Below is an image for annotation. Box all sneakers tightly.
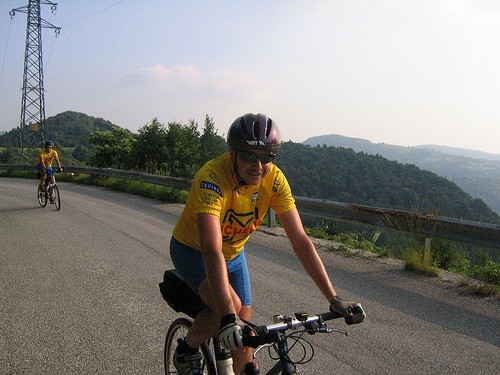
[39,183,46,197]
[172,338,205,374]
[50,198,56,204]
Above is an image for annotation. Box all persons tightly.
[37,141,63,205]
[170,113,367,375]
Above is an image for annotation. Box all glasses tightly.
[241,153,279,165]
[47,146,52,148]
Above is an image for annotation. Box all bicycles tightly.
[158,269,363,375]
[36,169,61,211]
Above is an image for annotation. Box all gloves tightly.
[58,168,62,172]
[42,168,48,173]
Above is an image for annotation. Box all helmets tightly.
[228,113,282,153]
[46,140,54,150]
